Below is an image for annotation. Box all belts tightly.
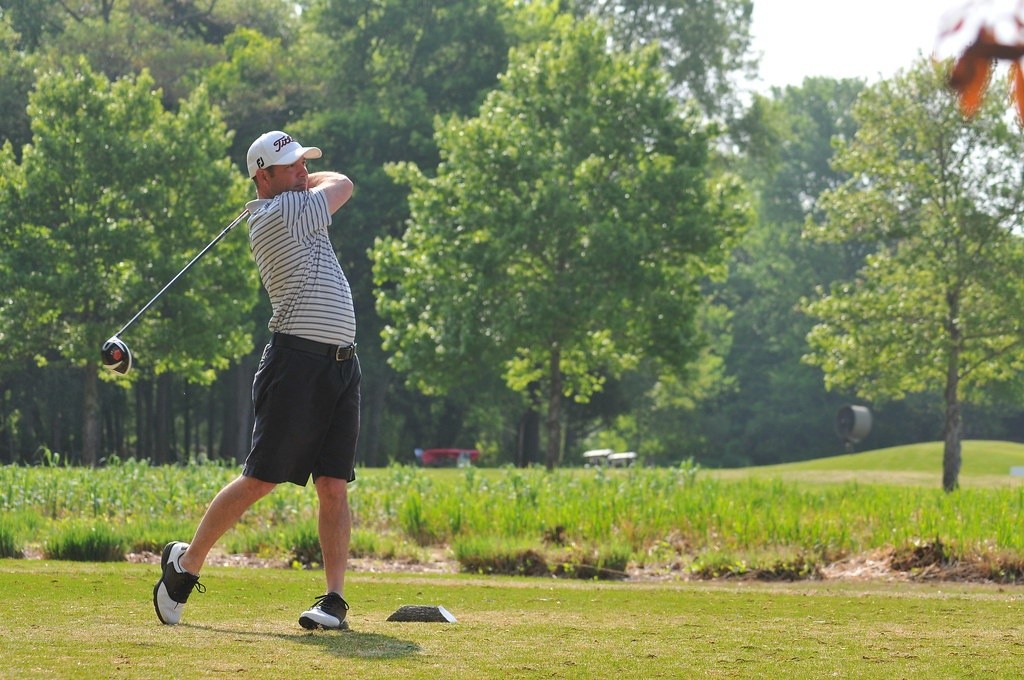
[269,333,358,361]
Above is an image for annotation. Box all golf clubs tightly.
[98,205,250,377]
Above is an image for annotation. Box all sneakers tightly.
[153,540,206,625]
[298,592,350,630]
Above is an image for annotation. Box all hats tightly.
[247,130,322,178]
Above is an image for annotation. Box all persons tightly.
[151,131,362,628]
